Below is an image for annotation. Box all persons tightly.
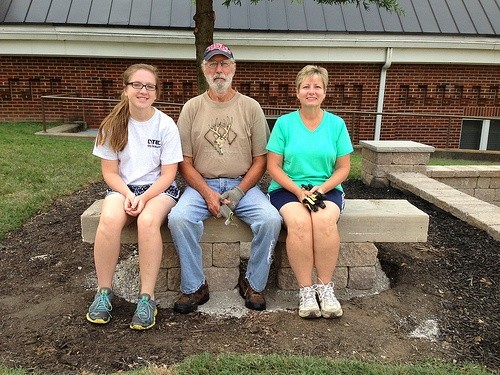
[266,64,353,317]
[167,43,284,313]
[86,64,183,330]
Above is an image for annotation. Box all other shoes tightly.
[239,276,266,310]
[172,281,211,312]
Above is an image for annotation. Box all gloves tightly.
[219,186,246,225]
[301,183,329,213]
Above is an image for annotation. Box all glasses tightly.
[124,81,158,92]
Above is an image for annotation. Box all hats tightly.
[202,41,233,61]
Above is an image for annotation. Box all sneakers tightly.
[86,288,113,326]
[316,283,344,319]
[130,297,158,331]
[298,284,321,320]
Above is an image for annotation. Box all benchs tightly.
[80,199,429,290]
